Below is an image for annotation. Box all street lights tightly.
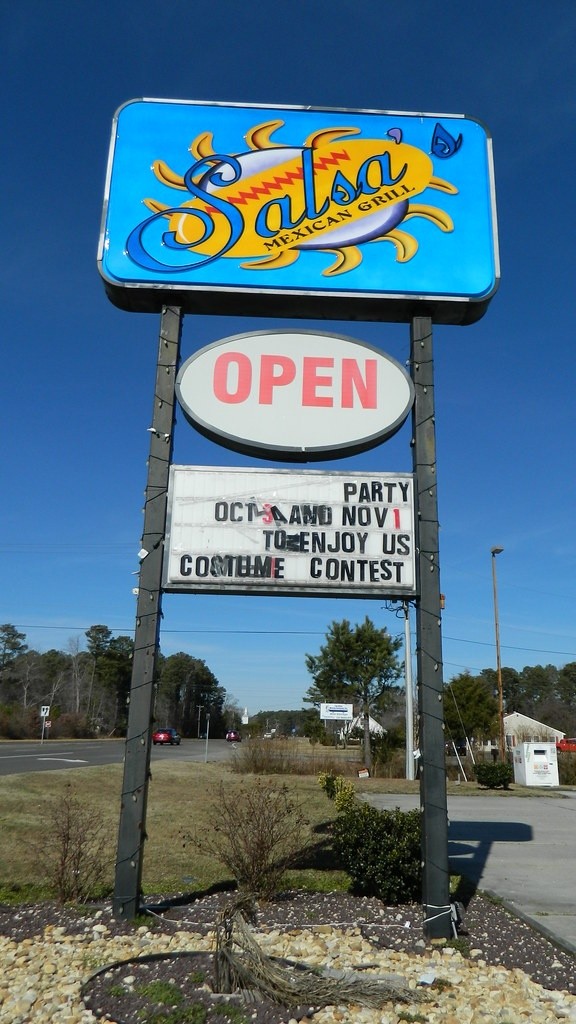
[490,544,510,790]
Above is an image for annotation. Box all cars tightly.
[445,739,466,756]
[556,738,576,753]
[225,730,242,743]
[152,727,182,745]
[264,732,273,740]
